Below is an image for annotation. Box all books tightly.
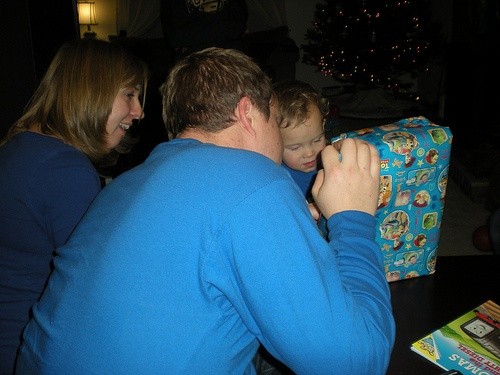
[410,299,500,375]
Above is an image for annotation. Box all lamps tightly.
[76,1,99,39]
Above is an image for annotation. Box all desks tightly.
[258,254,500,375]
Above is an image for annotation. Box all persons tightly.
[0,34,150,375]
[274,81,331,225]
[13,46,398,374]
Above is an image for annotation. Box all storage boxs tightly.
[316,115,453,284]
